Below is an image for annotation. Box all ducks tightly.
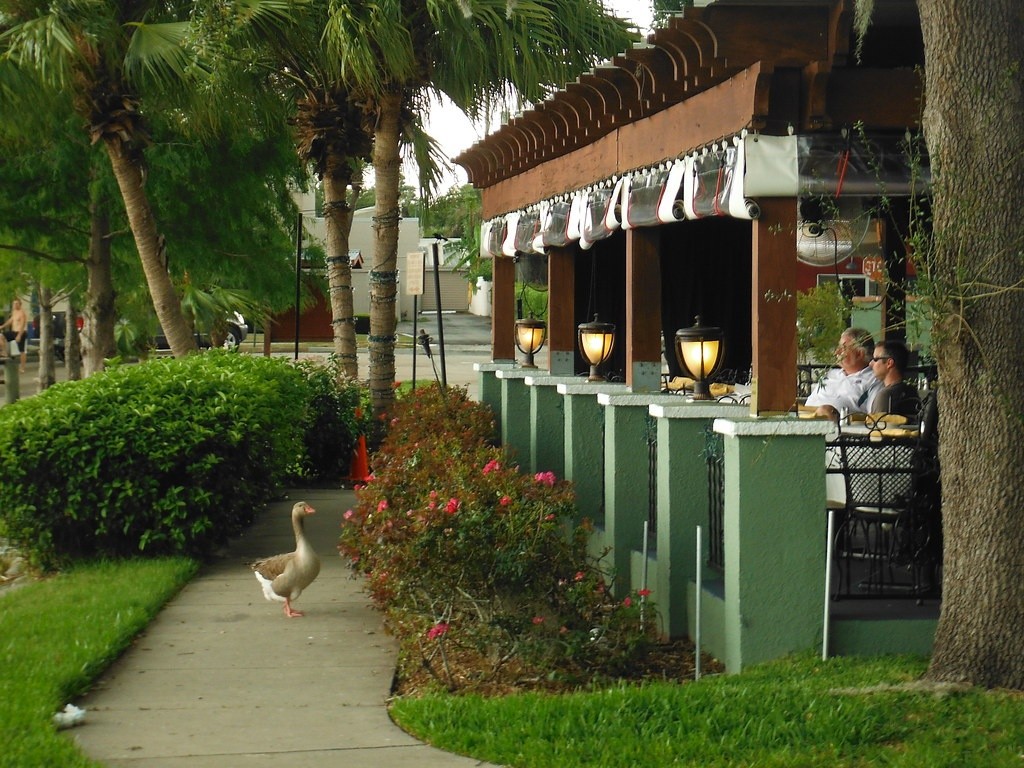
[246,501,321,618]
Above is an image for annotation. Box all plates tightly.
[864,421,903,429]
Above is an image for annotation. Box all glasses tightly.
[870,357,889,362]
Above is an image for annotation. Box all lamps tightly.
[514,312,547,368]
[675,314,726,401]
[577,313,616,380]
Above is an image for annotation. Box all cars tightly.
[27,298,247,363]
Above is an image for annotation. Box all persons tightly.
[805,327,885,416]
[0,298,28,372]
[869,339,921,425]
[53,314,66,361]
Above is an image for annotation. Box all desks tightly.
[826,421,913,559]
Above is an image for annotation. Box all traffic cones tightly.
[339,408,373,488]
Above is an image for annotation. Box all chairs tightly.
[831,410,931,606]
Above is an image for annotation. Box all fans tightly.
[797,149,870,266]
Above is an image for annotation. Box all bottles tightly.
[840,406,848,426]
